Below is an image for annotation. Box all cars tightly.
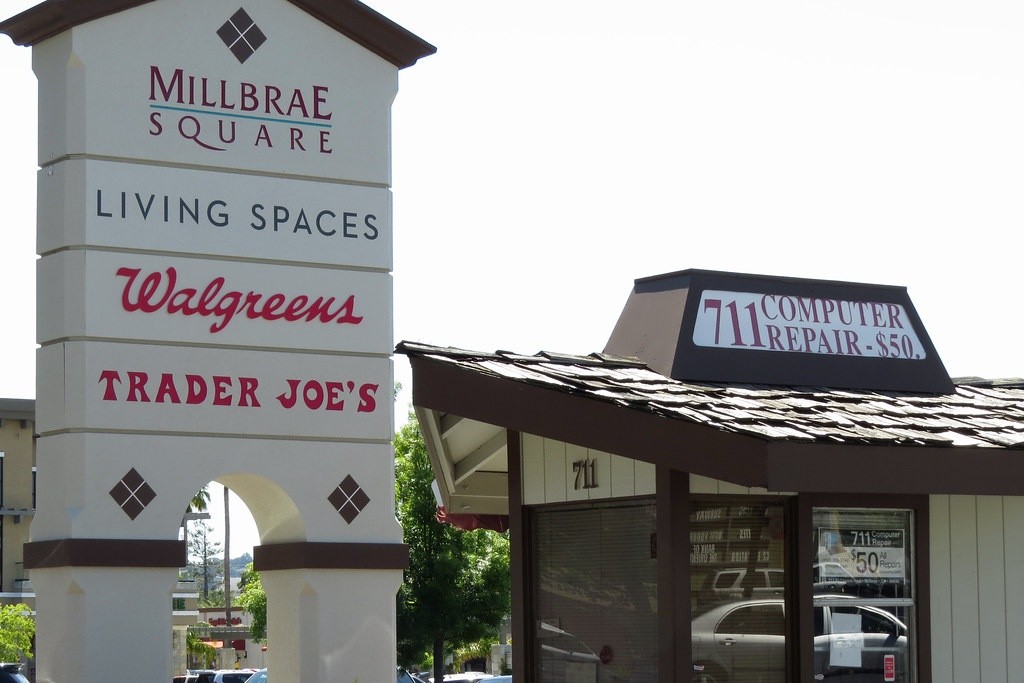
[172,665,267,683]
[686,597,786,683]
[697,566,785,603]
[813,594,909,683]
[813,562,862,594]
[395,663,512,683]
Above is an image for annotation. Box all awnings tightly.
[197,639,245,650]
